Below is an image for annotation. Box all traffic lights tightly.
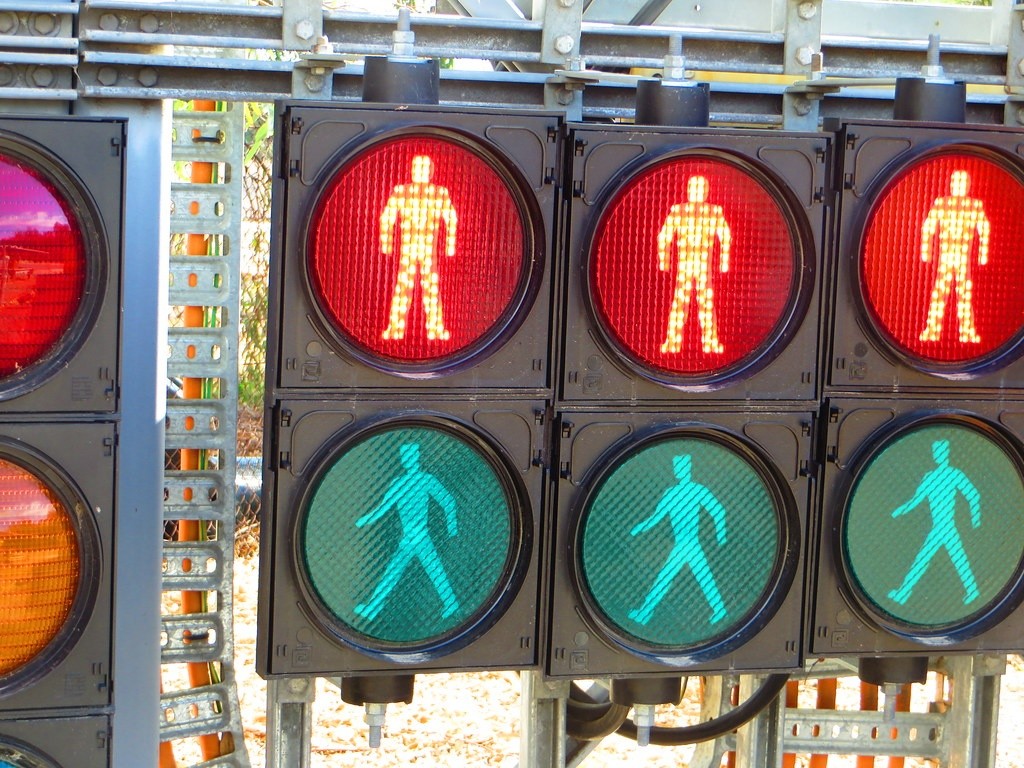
[0,113,131,768]
[254,57,1024,685]
